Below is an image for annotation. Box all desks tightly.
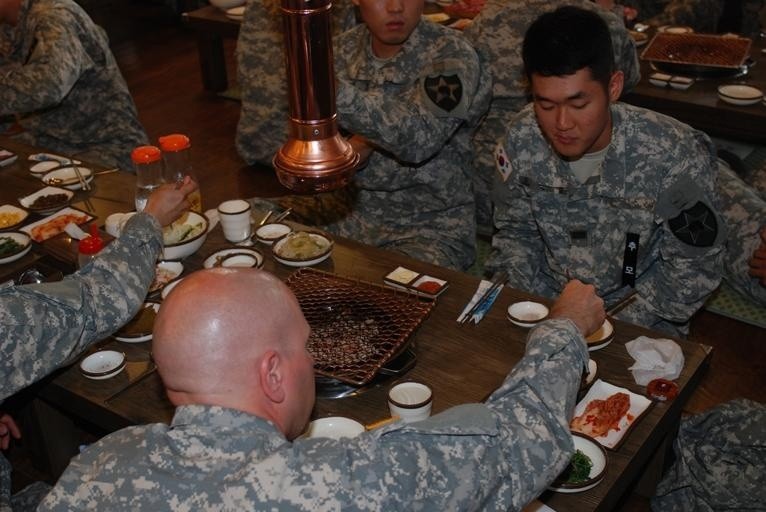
[0,126,715,511]
[624,39,766,155]
[183,3,239,96]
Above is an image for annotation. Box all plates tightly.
[226,7,245,20]
[78,348,128,381]
[0,148,97,264]
[383,266,449,298]
[506,301,551,328]
[630,33,649,47]
[567,377,658,452]
[424,13,474,31]
[662,26,694,35]
[306,417,368,440]
[717,85,764,106]
[649,71,696,90]
[583,317,615,352]
[544,429,608,492]
[580,356,601,389]
[106,206,333,343]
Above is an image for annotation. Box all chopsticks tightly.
[604,289,638,316]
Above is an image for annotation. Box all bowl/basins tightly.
[210,1,247,10]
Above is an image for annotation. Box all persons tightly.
[0,176,199,511]
[318,1,494,276]
[0,1,150,174]
[233,0,359,181]
[31,263,604,510]
[642,1,766,40]
[460,0,643,229]
[710,144,766,315]
[482,10,725,340]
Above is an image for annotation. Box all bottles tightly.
[130,146,166,213]
[158,133,202,215]
[77,221,103,276]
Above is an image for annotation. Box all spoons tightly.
[45,165,119,186]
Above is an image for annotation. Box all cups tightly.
[388,381,433,423]
[218,199,252,243]
